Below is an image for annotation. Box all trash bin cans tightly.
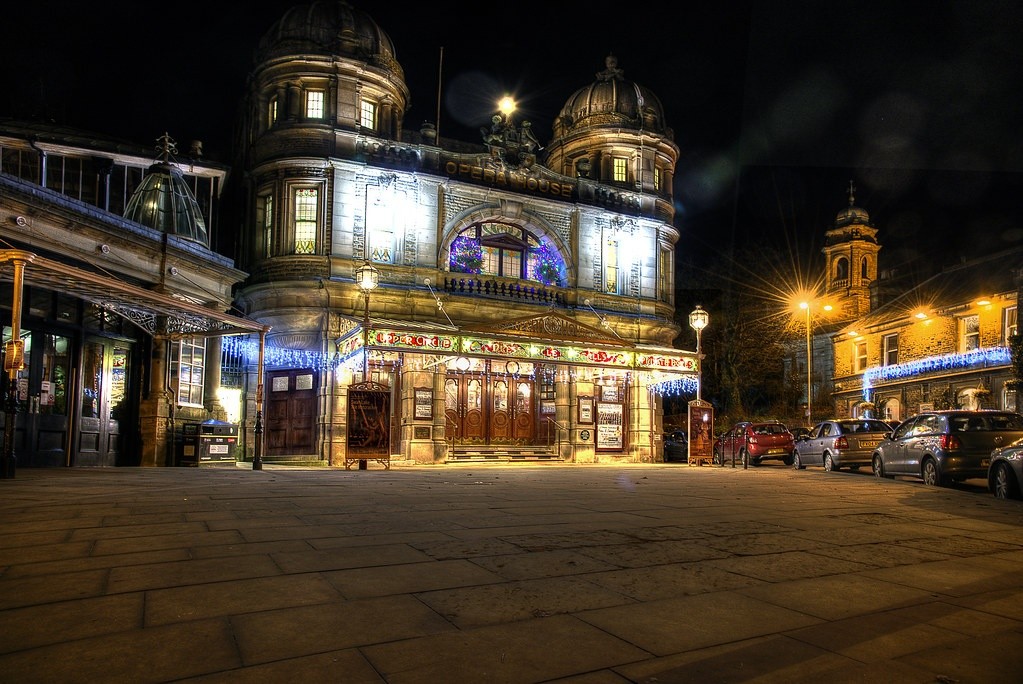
[181,422,238,469]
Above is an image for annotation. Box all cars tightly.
[788,426,825,441]
[983,436,1023,501]
[663,429,720,464]
[792,417,896,476]
[870,408,1023,490]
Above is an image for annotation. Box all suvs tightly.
[713,420,796,467]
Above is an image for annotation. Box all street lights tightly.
[689,304,710,401]
[796,300,834,427]
[353,258,379,469]
